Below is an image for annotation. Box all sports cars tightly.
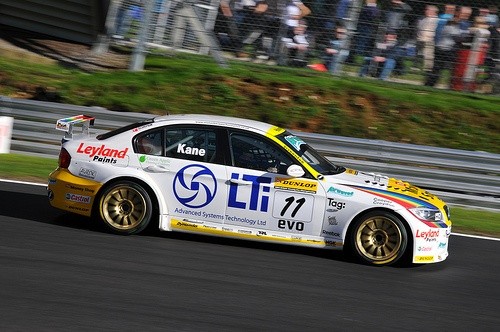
[46,113,452,266]
